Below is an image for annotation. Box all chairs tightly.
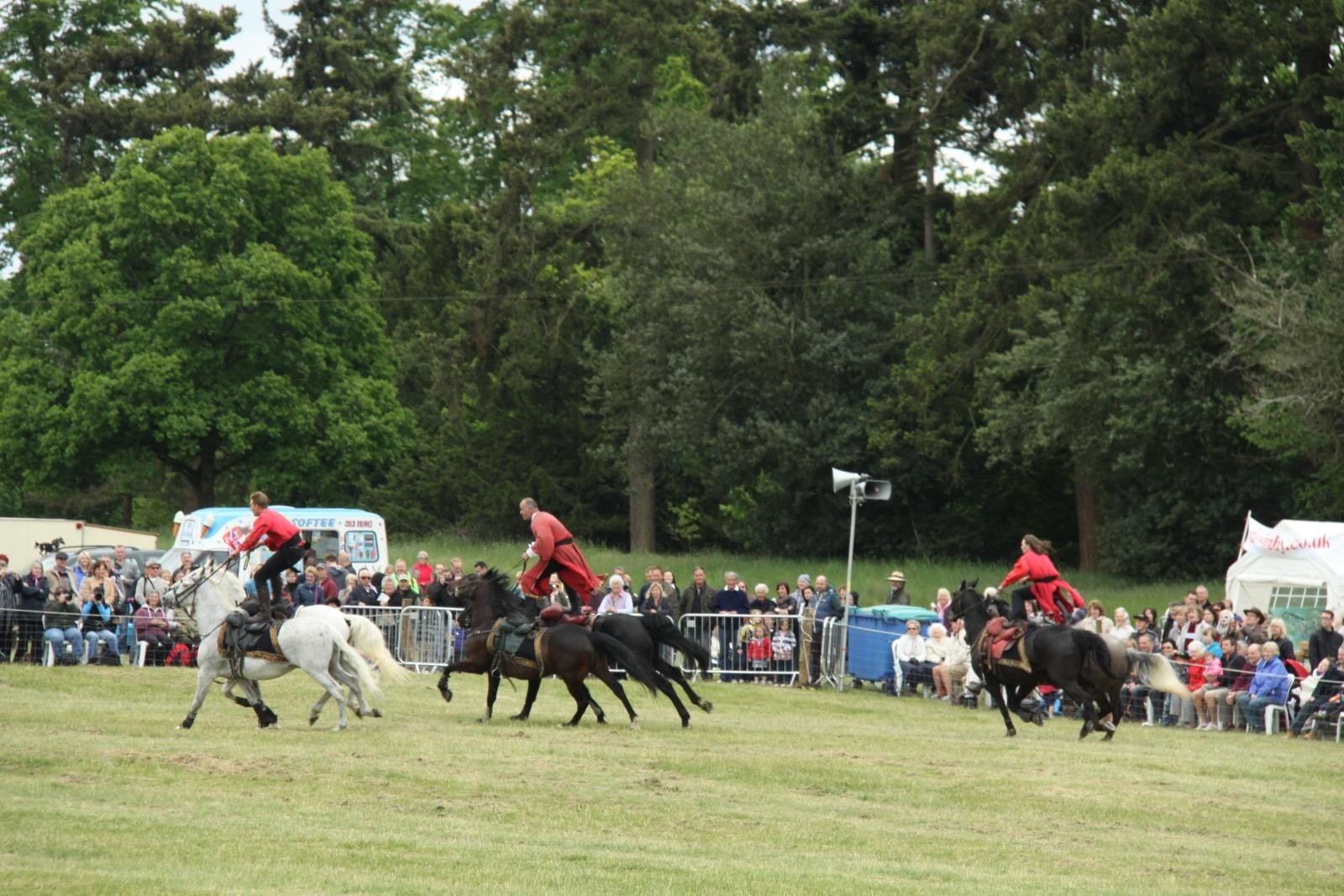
[43,613,173,666]
[1180,673,1344,743]
[892,636,974,701]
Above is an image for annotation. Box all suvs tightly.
[28,541,167,587]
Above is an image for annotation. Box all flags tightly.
[1243,516,1331,552]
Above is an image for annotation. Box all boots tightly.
[245,584,272,623]
[565,587,581,614]
[521,595,536,621]
[270,577,285,603]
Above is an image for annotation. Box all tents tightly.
[1225,519,1344,632]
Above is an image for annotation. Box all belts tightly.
[1033,577,1055,582]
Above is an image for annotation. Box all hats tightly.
[315,563,327,569]
[56,552,68,559]
[1244,607,1265,624]
[1132,613,1147,620]
[145,556,161,567]
[304,566,319,578]
[797,574,811,585]
[398,574,409,581]
[886,570,908,582]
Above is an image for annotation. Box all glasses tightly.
[1162,647,1170,649]
[907,628,917,630]
[359,576,371,580]
[152,566,159,569]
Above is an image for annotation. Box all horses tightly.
[943,578,1193,743]
[160,558,411,734]
[35,538,65,556]
[435,568,715,730]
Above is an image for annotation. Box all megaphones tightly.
[832,467,870,493]
[858,480,891,500]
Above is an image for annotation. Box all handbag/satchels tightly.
[375,609,397,626]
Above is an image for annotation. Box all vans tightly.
[158,504,389,603]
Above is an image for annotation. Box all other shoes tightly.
[1196,722,1217,731]
[941,696,952,701]
[1002,618,1022,628]
[932,694,940,698]
[1302,731,1318,739]
[1282,730,1296,738]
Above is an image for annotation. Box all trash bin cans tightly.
[845,604,942,695]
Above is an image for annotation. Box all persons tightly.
[0,492,1344,741]
